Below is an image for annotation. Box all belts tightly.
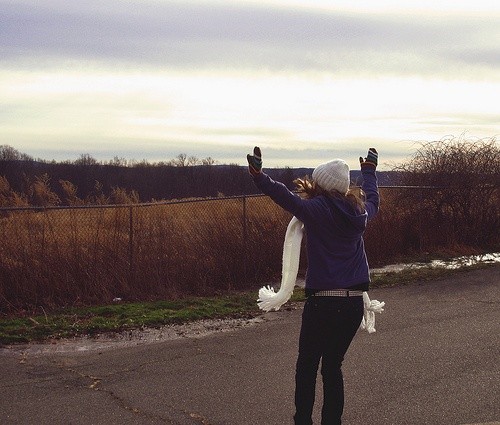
[312,290,364,297]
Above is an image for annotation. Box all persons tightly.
[247,146,386,425]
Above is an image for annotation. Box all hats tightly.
[312,160,356,194]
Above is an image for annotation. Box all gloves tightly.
[247,146,265,177]
[359,147,383,172]
